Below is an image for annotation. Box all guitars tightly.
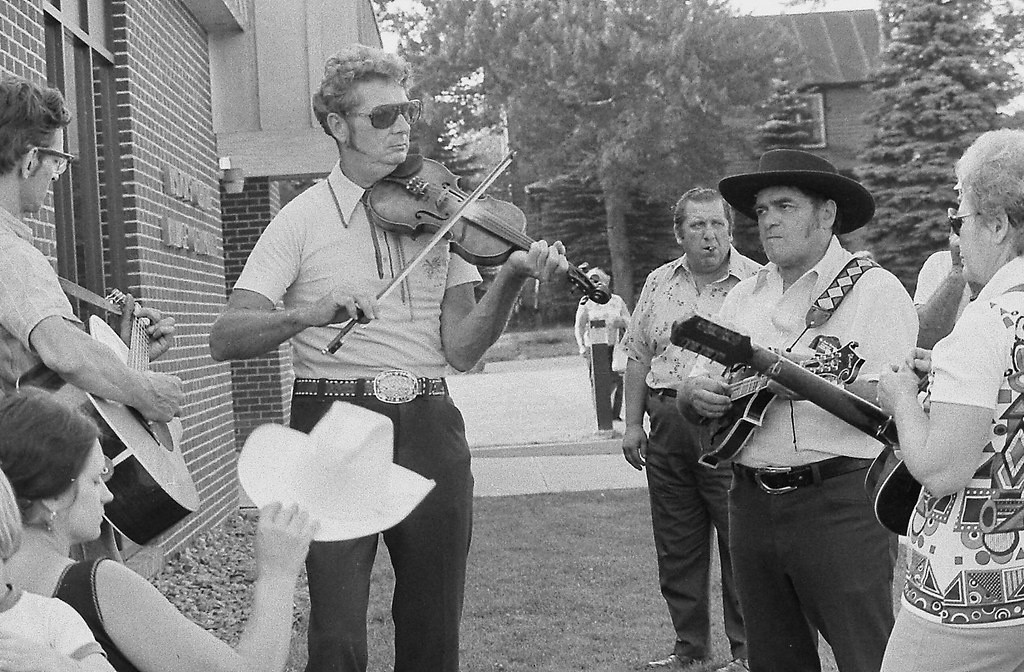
[668,312,933,537]
[15,287,202,549]
[698,338,867,473]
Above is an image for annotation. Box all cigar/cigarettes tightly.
[708,246,715,252]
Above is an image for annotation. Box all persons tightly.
[677,148,919,672]
[621,188,764,672]
[0,468,117,672]
[575,268,630,438]
[913,226,984,349]
[208,44,568,672]
[0,75,185,567]
[876,130,1024,672]
[0,386,314,672]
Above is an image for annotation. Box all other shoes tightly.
[716,659,749,672]
[649,653,713,669]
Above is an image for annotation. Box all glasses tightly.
[948,207,1018,237]
[330,99,423,130]
[23,145,74,174]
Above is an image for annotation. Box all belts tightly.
[293,370,447,404]
[731,455,876,495]
[649,387,678,398]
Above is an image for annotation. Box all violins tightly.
[364,155,616,306]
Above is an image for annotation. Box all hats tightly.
[717,148,875,235]
[238,401,436,542]
